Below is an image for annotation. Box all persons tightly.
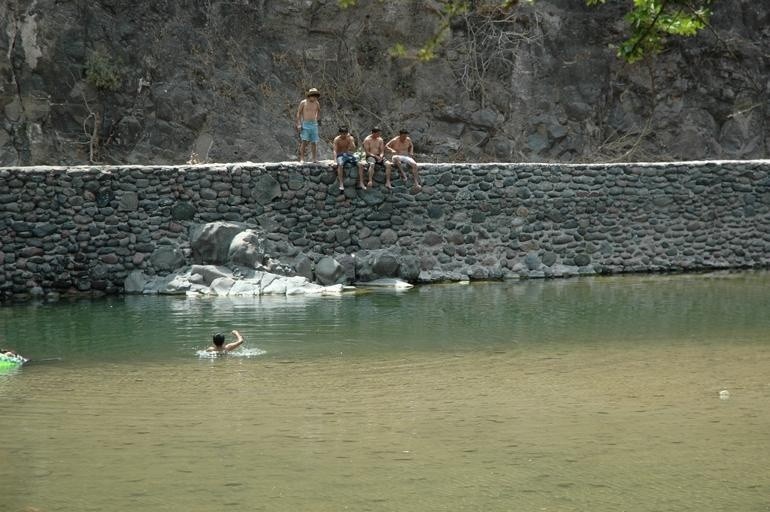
[362,127,394,189]
[205,328,244,354]
[296,88,323,165]
[332,126,367,192]
[385,129,423,189]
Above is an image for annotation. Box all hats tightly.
[305,88,320,97]
